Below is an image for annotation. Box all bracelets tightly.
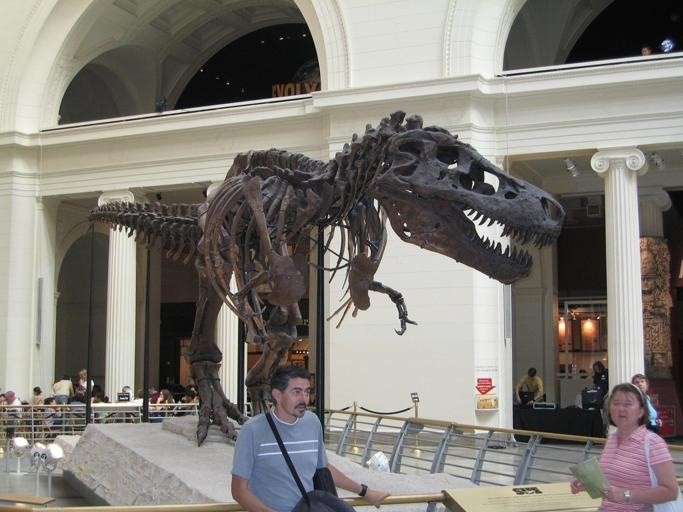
[359,483,368,496]
[533,399,536,402]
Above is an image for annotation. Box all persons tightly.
[641,46,652,56]
[230,365,390,512]
[631,373,661,434]
[588,360,608,402]
[570,383,678,512]
[1,366,202,443]
[515,367,545,408]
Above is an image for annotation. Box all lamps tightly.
[41,443,65,497]
[29,442,45,497]
[10,437,30,475]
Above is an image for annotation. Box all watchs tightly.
[623,487,632,502]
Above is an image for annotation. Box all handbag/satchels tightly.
[647,485,683,512]
